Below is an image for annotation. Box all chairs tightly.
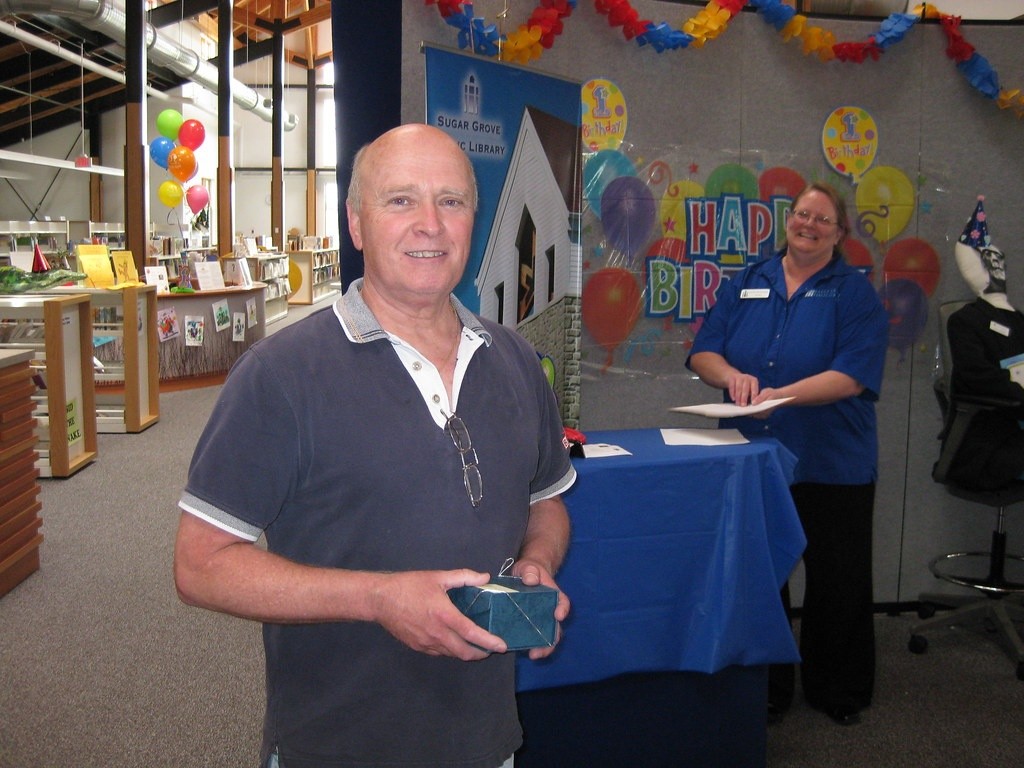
[884,299,1024,682]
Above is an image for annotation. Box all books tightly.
[0,235,340,351]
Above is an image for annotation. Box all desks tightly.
[510,425,810,768]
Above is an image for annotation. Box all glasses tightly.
[789,209,838,228]
[440,407,484,513]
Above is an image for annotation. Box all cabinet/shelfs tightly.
[0,219,339,601]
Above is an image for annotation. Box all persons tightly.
[931,239,1024,507]
[684,179,889,725]
[172,121,578,768]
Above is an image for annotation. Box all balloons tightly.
[582,148,942,354]
[149,108,209,215]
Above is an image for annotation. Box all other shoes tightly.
[827,703,860,726]
[769,692,791,715]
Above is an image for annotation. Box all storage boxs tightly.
[446,574,558,651]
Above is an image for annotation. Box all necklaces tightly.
[438,302,458,373]
